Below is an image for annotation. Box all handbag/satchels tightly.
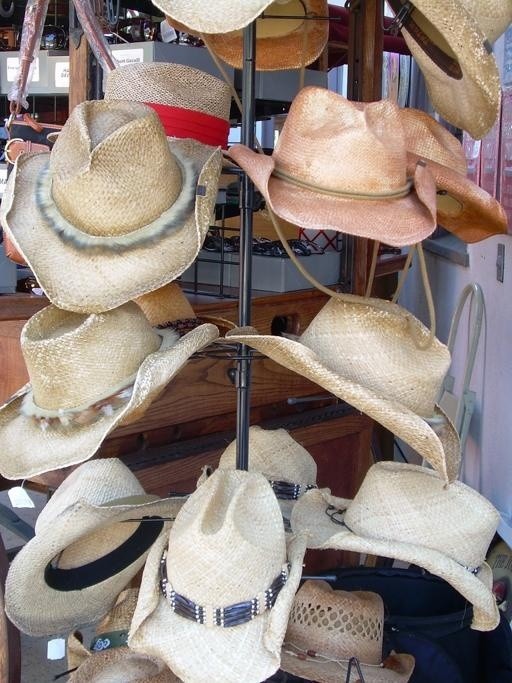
[4,0,117,266]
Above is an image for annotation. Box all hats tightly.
[1,299,219,480]
[48,61,242,168]
[400,106,508,243]
[128,469,309,682]
[224,292,461,485]
[4,458,190,638]
[198,425,331,540]
[227,84,437,245]
[68,588,141,678]
[66,645,183,682]
[388,603,511,682]
[130,281,239,341]
[1,98,222,313]
[281,579,416,682]
[151,0,329,71]
[385,0,512,140]
[291,459,501,632]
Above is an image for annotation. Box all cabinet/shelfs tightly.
[2,284,395,681]
